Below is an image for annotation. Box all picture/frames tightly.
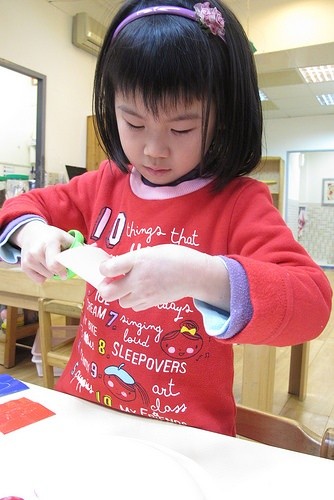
[322,178,334,206]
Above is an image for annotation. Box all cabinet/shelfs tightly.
[243,156,285,215]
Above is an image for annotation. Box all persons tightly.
[0,0,333,437]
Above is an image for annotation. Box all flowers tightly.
[193,2,226,36]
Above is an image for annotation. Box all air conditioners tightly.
[71,12,108,57]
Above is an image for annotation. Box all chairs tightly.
[38,298,83,389]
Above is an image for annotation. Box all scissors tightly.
[51,229,85,281]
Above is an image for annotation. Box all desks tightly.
[0,266,310,415]
[0,381,334,500]
